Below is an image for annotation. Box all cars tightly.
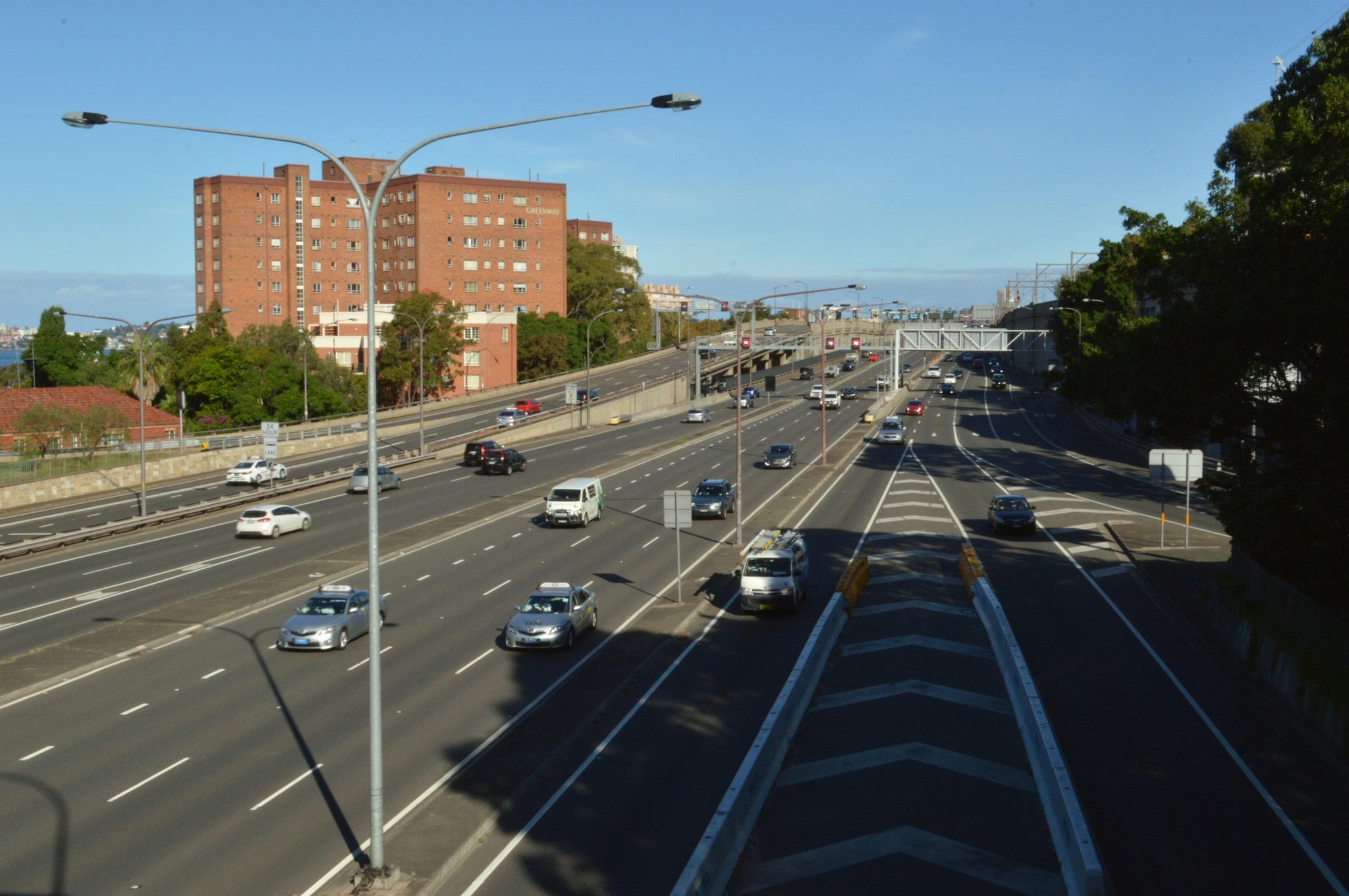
[276,583,389,652]
[347,464,402,492]
[742,386,759,399]
[234,503,312,540]
[226,455,289,486]
[577,389,600,406]
[733,394,754,409]
[691,326,1010,446]
[685,408,712,423]
[504,580,602,652]
[495,407,531,428]
[987,493,1037,536]
[763,443,798,469]
[514,399,543,414]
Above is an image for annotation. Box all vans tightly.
[543,477,605,528]
[738,529,811,615]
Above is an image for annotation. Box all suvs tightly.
[463,440,528,475]
[689,478,736,520]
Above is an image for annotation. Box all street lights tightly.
[303,317,358,424]
[357,308,493,456]
[59,90,708,877]
[620,284,869,540]
[586,309,623,430]
[58,307,232,518]
[751,300,904,465]
[1048,307,1082,401]
